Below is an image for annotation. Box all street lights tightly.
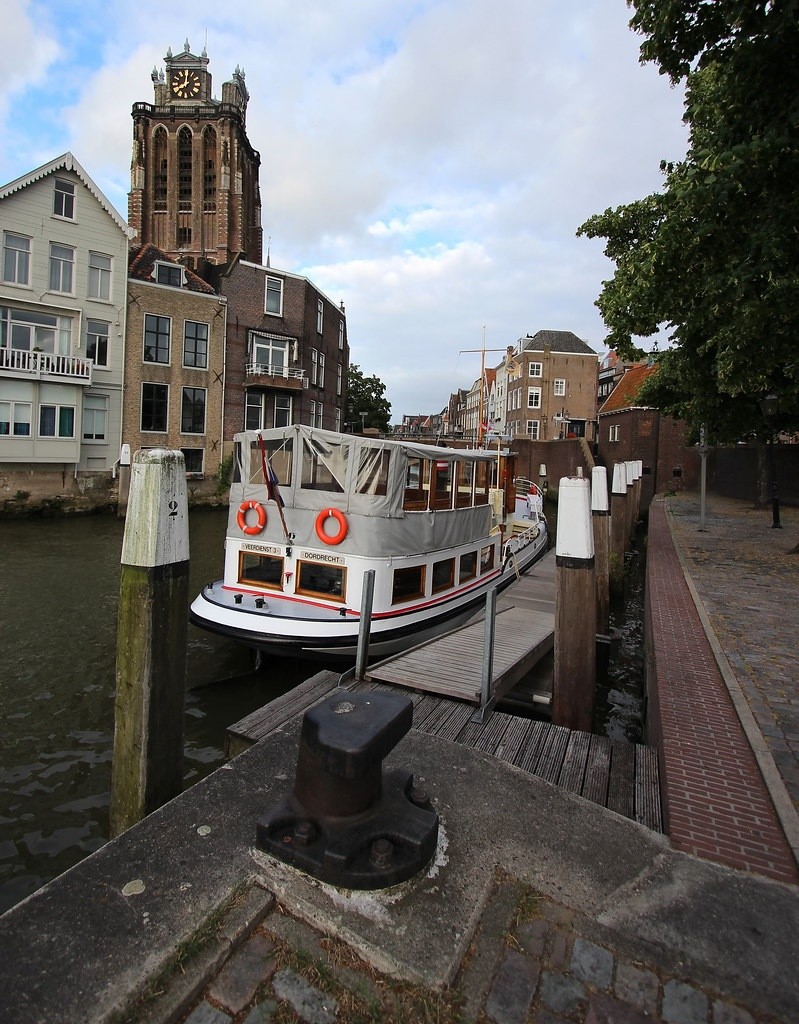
[755,393,782,528]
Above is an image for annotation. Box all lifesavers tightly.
[316,508,348,546]
[237,499,266,536]
[529,486,537,496]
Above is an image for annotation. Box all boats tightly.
[190,423,550,666]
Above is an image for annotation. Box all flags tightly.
[258,433,286,509]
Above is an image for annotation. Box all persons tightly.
[559,429,578,439]
